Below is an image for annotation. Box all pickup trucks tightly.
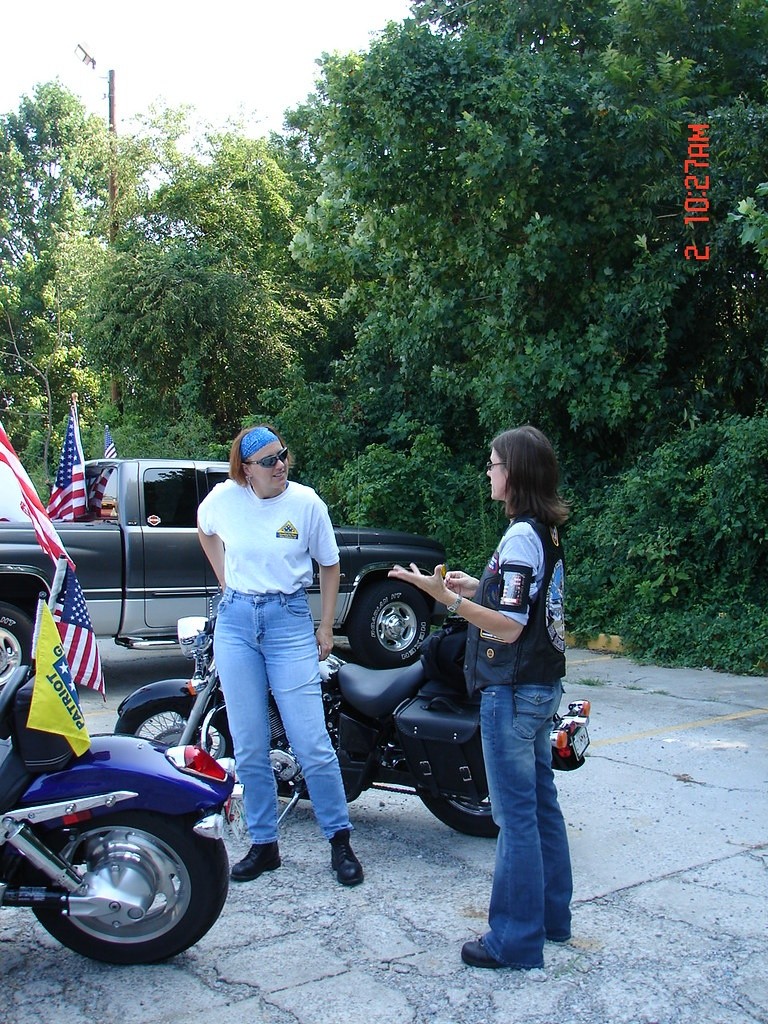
[0,457,449,695]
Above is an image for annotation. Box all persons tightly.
[197,424,364,882]
[388,424,573,969]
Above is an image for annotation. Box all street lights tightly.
[77,44,121,418]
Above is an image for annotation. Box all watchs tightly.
[446,594,462,612]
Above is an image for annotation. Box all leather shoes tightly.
[461,935,507,968]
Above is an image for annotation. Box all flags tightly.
[86,467,114,517]
[0,423,77,573]
[104,429,118,459]
[26,600,92,757]
[46,406,89,523]
[47,557,106,703]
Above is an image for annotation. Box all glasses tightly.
[242,446,288,468]
[485,460,508,471]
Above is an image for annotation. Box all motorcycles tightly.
[0,660,239,967]
[111,586,592,839]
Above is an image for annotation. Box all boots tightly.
[230,840,281,880]
[329,828,366,885]
